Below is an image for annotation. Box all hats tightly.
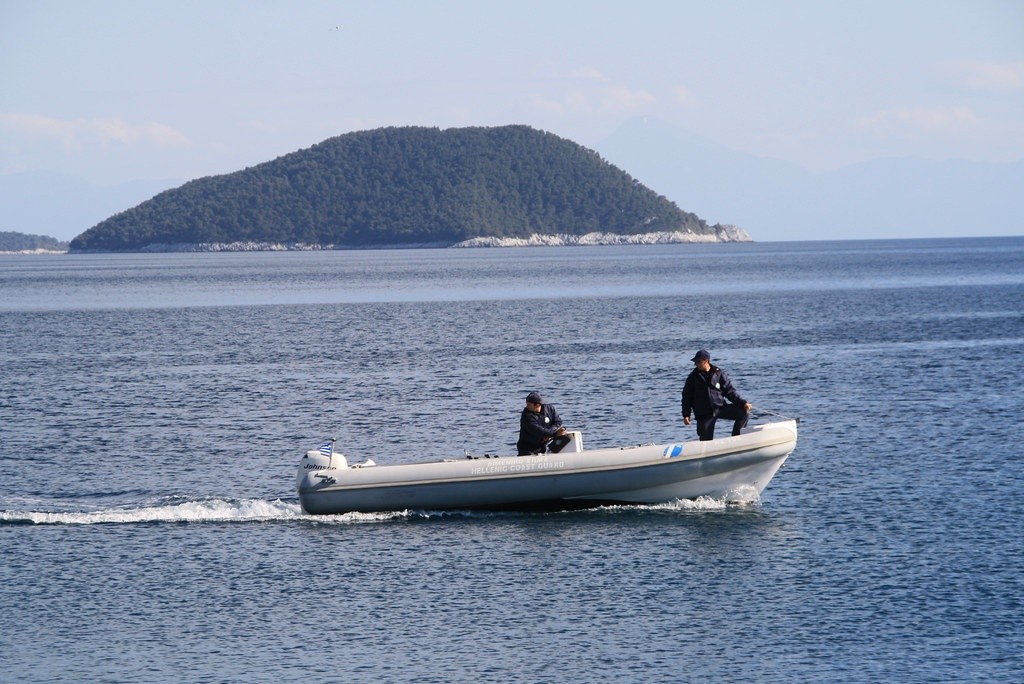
[526,392,541,402]
[691,349,710,362]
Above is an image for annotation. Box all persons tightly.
[516,393,567,456]
[681,350,752,441]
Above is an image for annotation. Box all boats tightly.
[293,416,801,523]
